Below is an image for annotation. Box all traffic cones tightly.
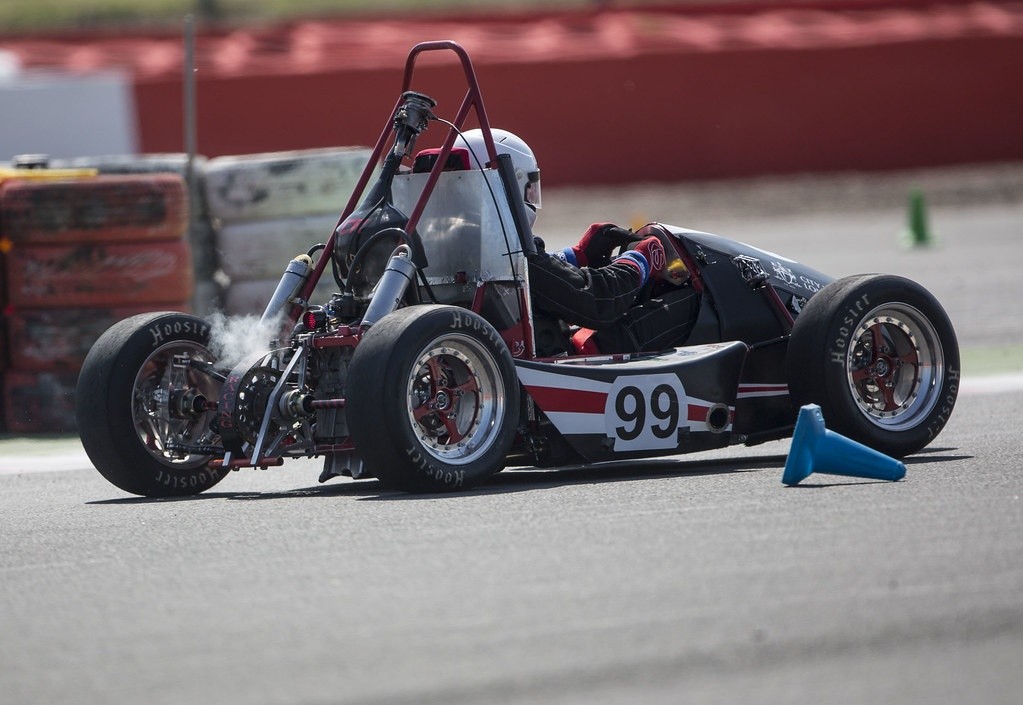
[901,189,937,252]
[782,403,907,487]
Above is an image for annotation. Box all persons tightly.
[440,128,701,358]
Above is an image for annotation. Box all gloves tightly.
[627,234,666,287]
[563,222,620,269]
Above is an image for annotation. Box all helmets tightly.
[441,127,542,229]
